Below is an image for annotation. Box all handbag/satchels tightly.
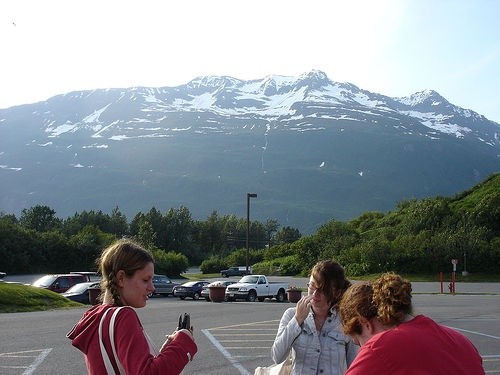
[255,353,293,375]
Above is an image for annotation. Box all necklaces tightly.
[315,318,323,329]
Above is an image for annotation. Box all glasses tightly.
[306,283,329,295]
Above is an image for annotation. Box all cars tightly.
[60,282,102,304]
[173,280,211,300]
[200,280,237,302]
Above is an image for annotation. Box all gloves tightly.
[178,312,194,336]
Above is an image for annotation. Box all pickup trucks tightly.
[220,267,250,278]
[31,273,89,293]
[225,274,288,302]
[147,275,182,298]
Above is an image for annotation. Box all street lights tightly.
[245,193,258,276]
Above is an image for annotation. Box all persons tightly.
[272,260,361,375]
[66,240,198,375]
[338,272,485,375]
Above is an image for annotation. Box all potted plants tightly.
[203,283,227,303]
[287,285,303,303]
